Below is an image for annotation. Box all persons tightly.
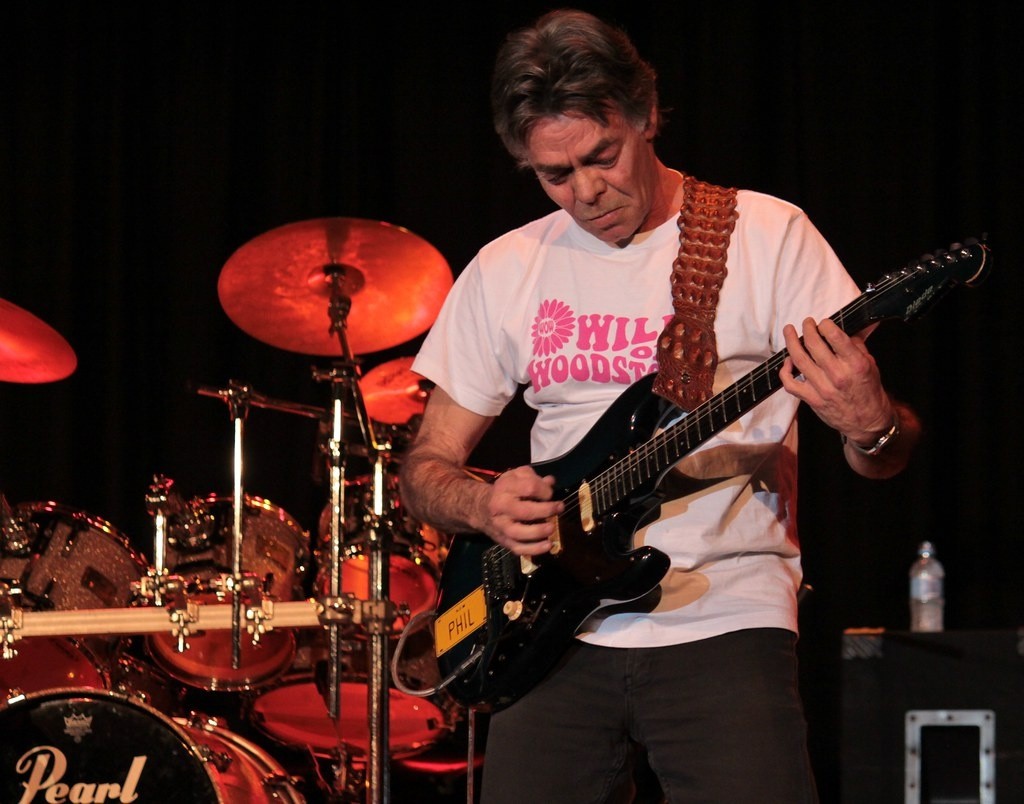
[398,10,921,804]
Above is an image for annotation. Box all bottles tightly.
[909,541,946,632]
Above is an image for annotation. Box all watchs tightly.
[847,406,902,458]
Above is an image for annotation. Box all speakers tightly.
[838,627,1024,804]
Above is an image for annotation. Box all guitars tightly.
[428,228,993,710]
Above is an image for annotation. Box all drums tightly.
[315,474,446,641]
[145,492,312,692]
[243,631,456,764]
[0,498,148,711]
[1,684,308,803]
[400,703,491,772]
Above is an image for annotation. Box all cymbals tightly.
[360,357,433,424]
[0,297,78,384]
[218,215,454,357]
